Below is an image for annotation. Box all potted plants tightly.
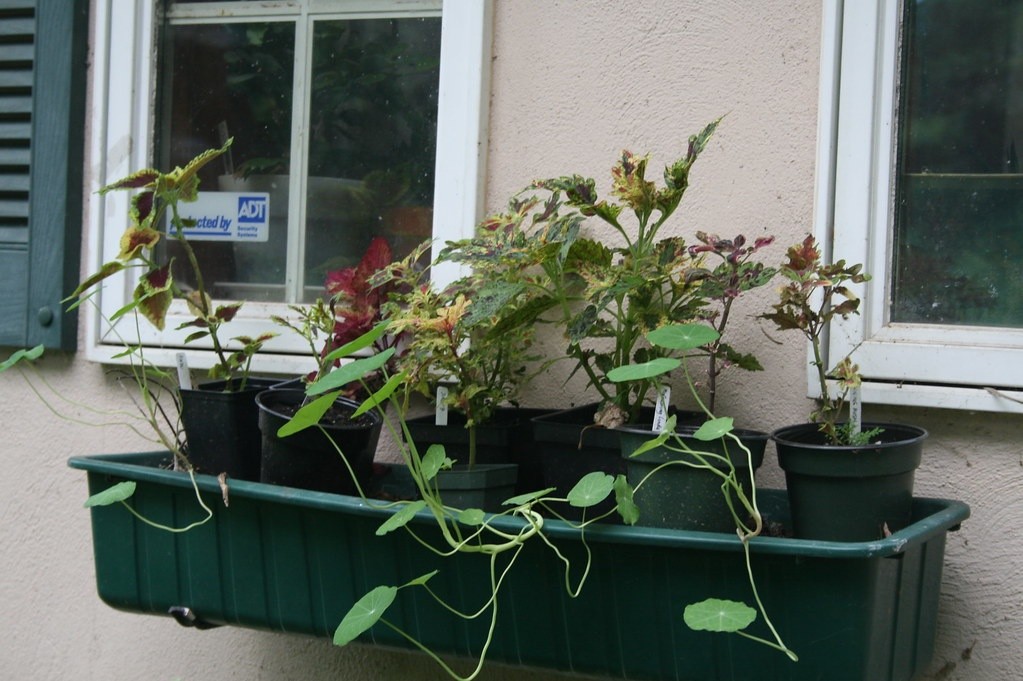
[0,286,971,681]
[255,240,395,496]
[769,236,929,546]
[619,232,769,534]
[364,238,544,514]
[214,115,381,296]
[61,136,288,526]
[429,113,733,522]
